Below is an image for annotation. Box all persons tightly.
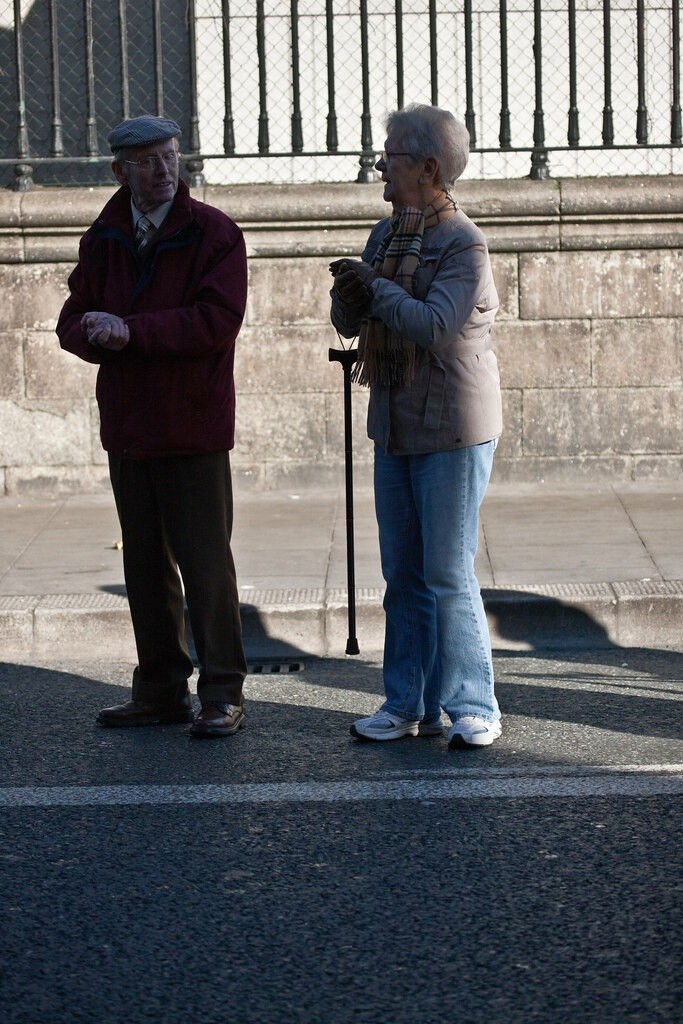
[55,115,247,735]
[329,106,504,748]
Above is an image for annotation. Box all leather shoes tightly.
[96,692,194,726]
[195,702,244,735]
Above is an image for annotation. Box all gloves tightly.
[328,259,381,287]
[331,262,370,308]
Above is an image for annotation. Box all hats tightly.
[108,115,181,154]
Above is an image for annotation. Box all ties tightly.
[133,217,151,261]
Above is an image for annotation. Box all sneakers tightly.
[448,715,502,745]
[350,709,444,740]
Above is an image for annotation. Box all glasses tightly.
[122,150,183,168]
[380,151,410,162]
